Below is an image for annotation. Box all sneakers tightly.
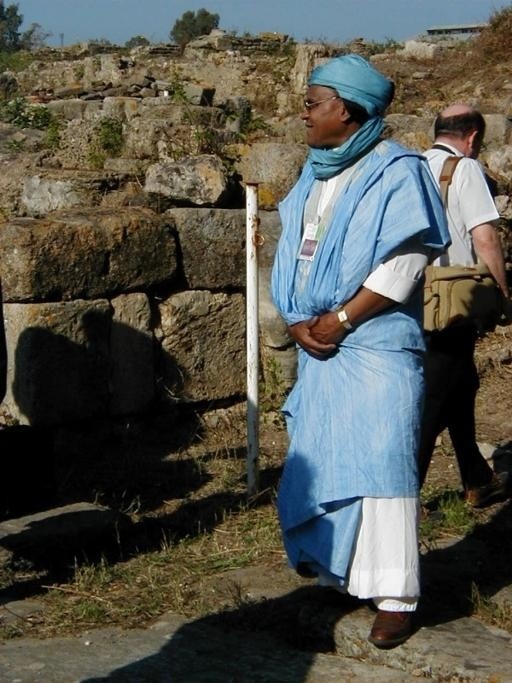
[466,471,511,507]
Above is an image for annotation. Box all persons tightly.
[417,104,509,508]
[269,53,453,646]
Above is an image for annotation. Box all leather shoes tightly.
[368,607,414,646]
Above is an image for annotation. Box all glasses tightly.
[302,94,338,110]
[478,139,486,153]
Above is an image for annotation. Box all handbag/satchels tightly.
[413,260,503,335]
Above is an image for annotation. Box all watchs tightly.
[334,306,353,331]
